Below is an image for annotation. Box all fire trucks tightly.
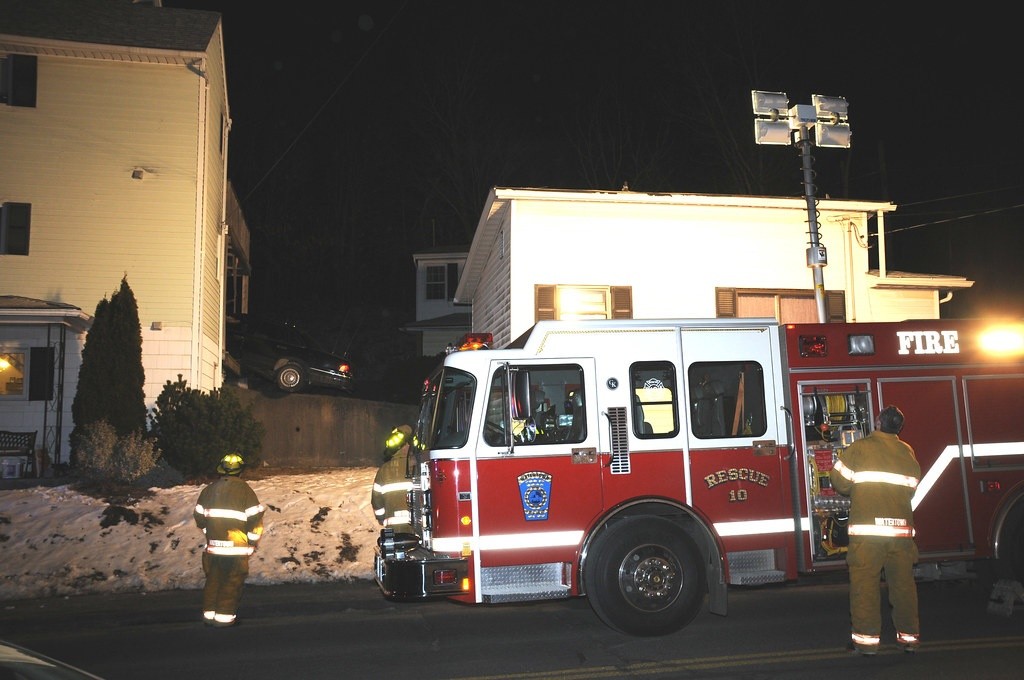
[372,311,1024,638]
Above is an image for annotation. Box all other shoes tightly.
[898,643,920,651]
[204,618,241,628]
[848,639,880,654]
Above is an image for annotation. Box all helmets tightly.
[217,453,245,474]
[383,425,413,458]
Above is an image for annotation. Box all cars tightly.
[225,308,369,398]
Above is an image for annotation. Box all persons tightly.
[829,404,920,654]
[192,454,264,627]
[372,425,419,537]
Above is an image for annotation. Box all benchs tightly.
[0,430,38,478]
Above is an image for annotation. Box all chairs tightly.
[635,394,645,436]
[691,380,726,436]
[572,389,585,441]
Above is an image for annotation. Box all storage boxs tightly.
[2,460,21,479]
